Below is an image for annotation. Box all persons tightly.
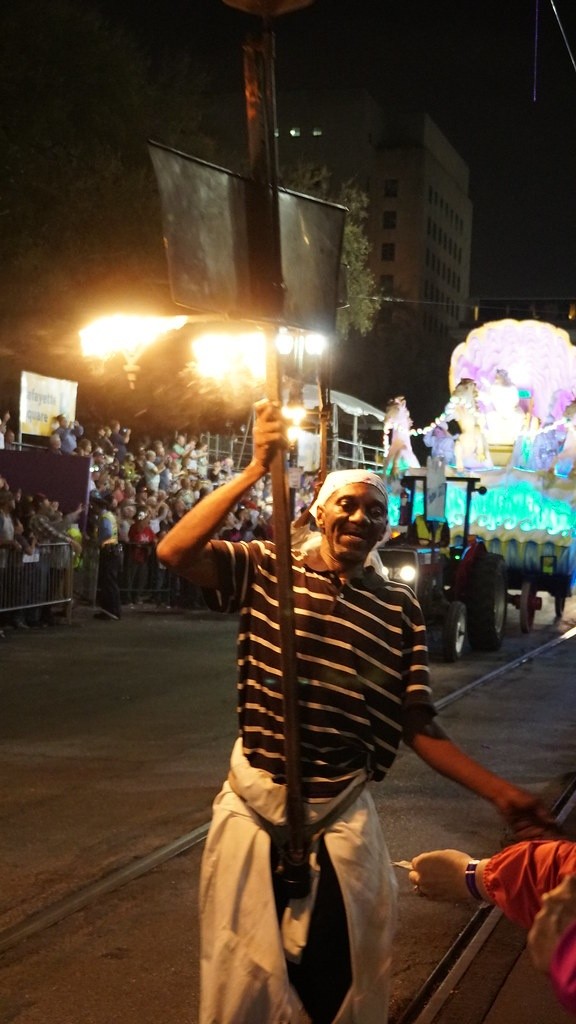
[407,840,576,1024]
[156,399,556,1024]
[0,409,318,638]
[382,369,576,485]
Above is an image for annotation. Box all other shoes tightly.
[15,621,32,632]
[156,602,170,609]
[101,608,121,620]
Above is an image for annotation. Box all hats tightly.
[136,487,149,492]
[0,491,17,503]
[31,493,49,507]
[119,499,139,508]
[90,498,109,505]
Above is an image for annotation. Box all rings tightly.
[415,886,424,897]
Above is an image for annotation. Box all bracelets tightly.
[465,857,484,901]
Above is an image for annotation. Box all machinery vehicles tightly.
[367,476,508,664]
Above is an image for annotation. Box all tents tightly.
[284,381,386,469]
